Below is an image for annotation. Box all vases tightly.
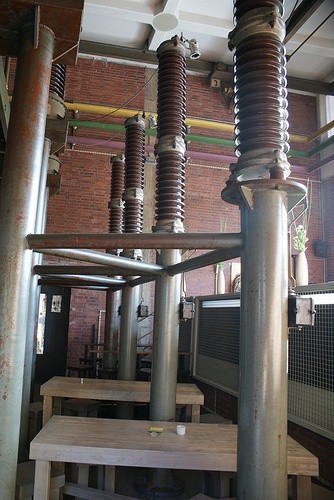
[295,253,308,286]
[217,270,225,294]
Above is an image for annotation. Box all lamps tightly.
[147,114,157,127]
[181,32,201,60]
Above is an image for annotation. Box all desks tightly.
[40,376,204,427]
[90,350,192,355]
[29,415,319,500]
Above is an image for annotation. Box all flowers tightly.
[293,225,309,252]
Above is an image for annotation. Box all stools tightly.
[14,357,237,500]
